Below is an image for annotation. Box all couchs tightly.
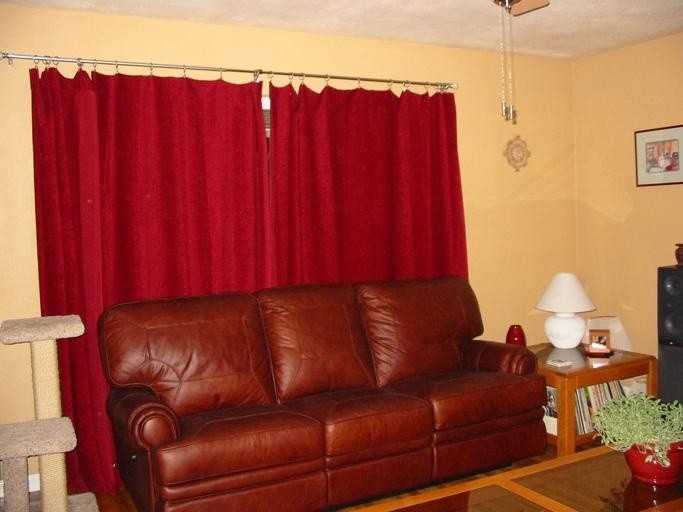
[93,274,549,512]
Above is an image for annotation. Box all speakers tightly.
[658,266,683,405]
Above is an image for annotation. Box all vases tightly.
[504,324,526,348]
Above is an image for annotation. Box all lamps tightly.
[532,270,598,351]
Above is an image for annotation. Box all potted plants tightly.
[591,390,683,487]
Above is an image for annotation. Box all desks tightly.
[352,442,682,511]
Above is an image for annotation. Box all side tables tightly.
[519,341,661,457]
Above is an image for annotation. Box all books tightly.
[539,379,630,438]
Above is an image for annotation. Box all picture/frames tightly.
[632,124,682,188]
